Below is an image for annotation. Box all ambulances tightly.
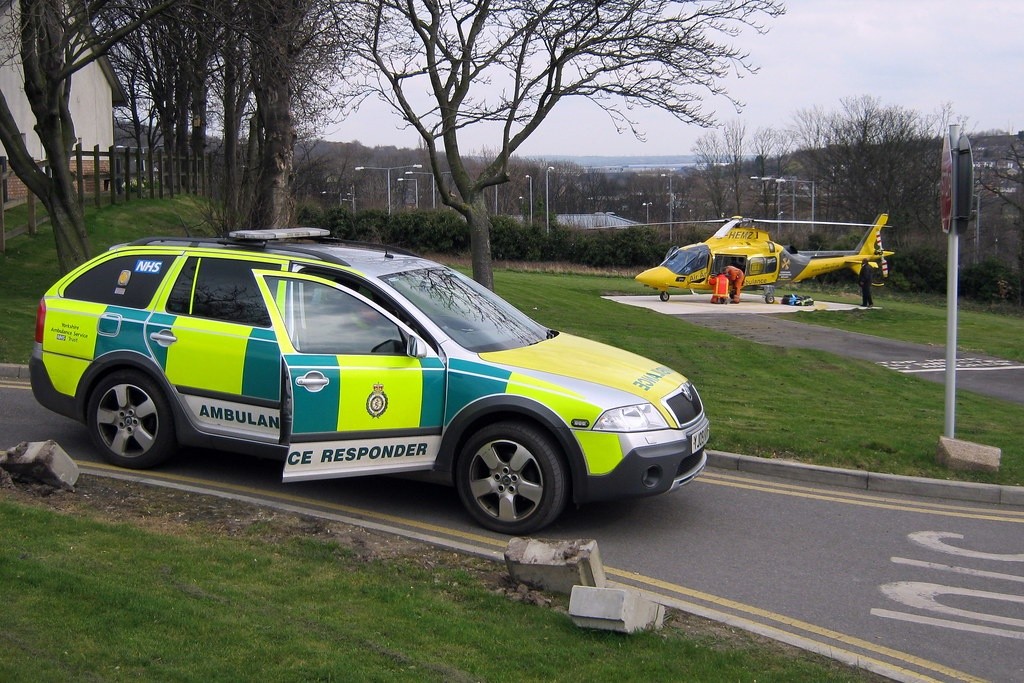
[29,227,710,538]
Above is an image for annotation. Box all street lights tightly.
[776,179,814,232]
[398,178,418,208]
[661,174,672,240]
[750,177,781,234]
[643,202,652,223]
[405,172,435,210]
[526,175,532,223]
[762,177,795,230]
[355,165,423,215]
[546,167,554,235]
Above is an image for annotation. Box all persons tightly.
[722,266,745,304]
[857,258,875,307]
[307,276,382,352]
[708,267,730,304]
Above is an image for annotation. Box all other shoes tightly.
[716,298,722,304]
[730,300,740,304]
[868,303,873,307]
[725,298,728,305]
[861,304,868,307]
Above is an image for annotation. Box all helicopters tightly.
[603,213,896,304]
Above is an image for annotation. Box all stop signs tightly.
[939,135,953,232]
